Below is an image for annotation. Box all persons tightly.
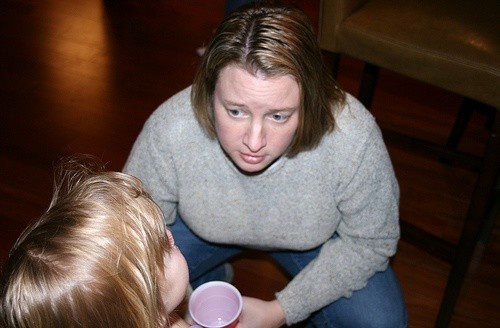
[119,4,408,327]
[1,161,208,328]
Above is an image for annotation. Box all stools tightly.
[315,1,500,328]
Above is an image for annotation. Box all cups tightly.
[189,280,242,328]
